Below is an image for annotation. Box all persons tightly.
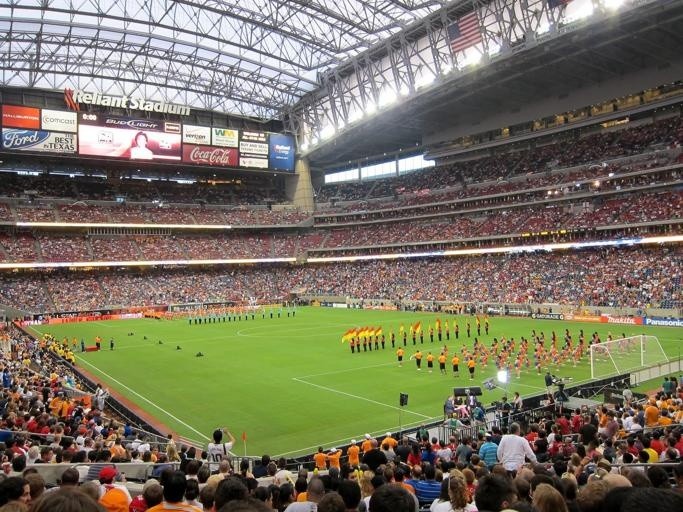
[1,106,682,324]
[1,316,683,512]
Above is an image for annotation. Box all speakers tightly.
[400,393,408,406]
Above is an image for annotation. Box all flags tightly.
[400,316,489,334]
[441,12,486,55]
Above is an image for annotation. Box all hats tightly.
[350,439,356,445]
[330,447,336,452]
[364,434,370,439]
[98,467,116,481]
[385,431,391,436]
[100,488,129,512]
